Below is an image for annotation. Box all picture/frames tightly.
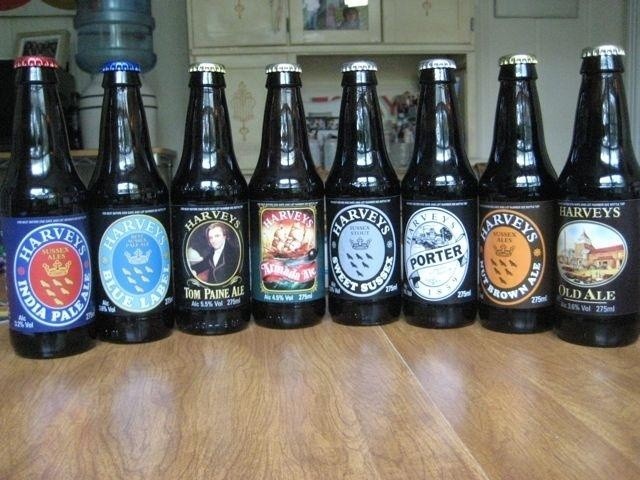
[290,0,381,44]
[13,30,70,70]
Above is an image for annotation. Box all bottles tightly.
[400,57,476,330]
[324,58,399,327]
[0,54,94,361]
[476,53,558,335]
[557,45,640,350]
[87,59,176,346]
[249,61,326,330]
[170,61,251,335]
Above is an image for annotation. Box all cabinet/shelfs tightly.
[382,2,474,48]
[187,1,289,50]
[193,55,297,184]
[0,59,76,151]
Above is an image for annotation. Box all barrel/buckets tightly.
[73,0,156,71]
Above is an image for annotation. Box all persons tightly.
[191,222,238,282]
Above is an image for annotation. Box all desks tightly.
[0,147,177,190]
